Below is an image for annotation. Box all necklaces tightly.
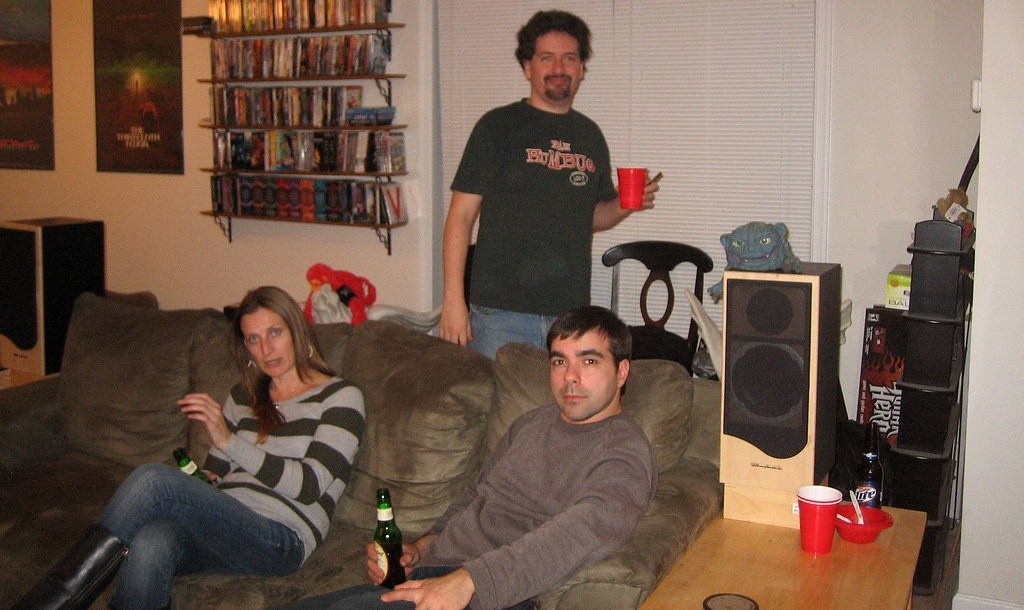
[276,306,658,610]
[275,382,301,409]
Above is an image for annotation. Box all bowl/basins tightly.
[834,504,894,544]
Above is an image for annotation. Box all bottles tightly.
[173,447,213,486]
[856,422,884,510]
[374,488,407,590]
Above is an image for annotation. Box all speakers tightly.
[0,216,105,375]
[720,262,843,530]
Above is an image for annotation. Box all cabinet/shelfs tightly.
[189,1,409,255]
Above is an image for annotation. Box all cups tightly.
[797,485,843,554]
[616,167,647,210]
[290,130,314,172]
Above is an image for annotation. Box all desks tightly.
[638,496,926,609]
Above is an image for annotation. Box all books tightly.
[209,0,410,224]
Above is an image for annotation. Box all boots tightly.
[11,524,124,610]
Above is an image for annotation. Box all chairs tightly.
[601,241,713,375]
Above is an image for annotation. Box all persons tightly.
[11,286,366,610]
[439,9,658,360]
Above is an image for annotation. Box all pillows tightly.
[59,293,696,540]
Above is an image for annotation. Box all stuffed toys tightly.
[303,264,377,323]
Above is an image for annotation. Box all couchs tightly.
[0,291,723,610]
